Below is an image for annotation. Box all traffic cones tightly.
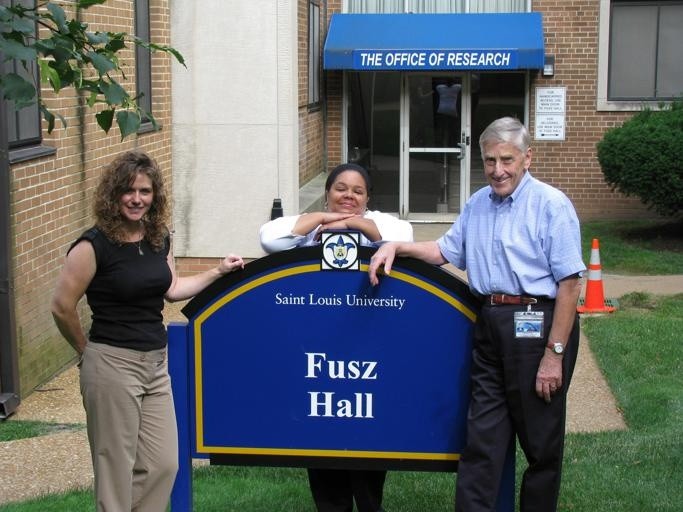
[578,238,618,315]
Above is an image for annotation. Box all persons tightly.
[414,73,483,164]
[258,162,413,512]
[49,150,246,512]
[367,114,588,512]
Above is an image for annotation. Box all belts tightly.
[480,293,538,306]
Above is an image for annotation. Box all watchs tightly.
[545,339,568,356]
